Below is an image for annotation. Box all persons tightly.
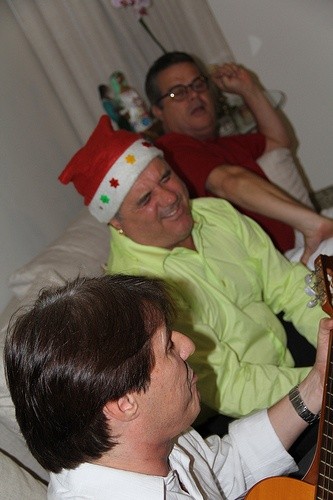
[144,51,333,266]
[3,274,332,500]
[56,114,332,464]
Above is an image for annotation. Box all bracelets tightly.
[288,385,319,426]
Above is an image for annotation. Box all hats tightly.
[58,115,163,223]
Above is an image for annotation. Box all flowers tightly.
[107,0,169,55]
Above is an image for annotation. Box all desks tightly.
[210,88,320,235]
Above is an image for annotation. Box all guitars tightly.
[235,254,332,500]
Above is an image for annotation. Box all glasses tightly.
[155,77,210,103]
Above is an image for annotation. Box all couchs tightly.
[0,218,113,500]
